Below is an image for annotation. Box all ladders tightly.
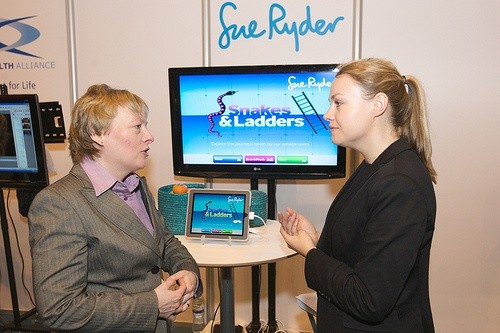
[292,92,328,134]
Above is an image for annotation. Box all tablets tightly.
[185,189,251,241]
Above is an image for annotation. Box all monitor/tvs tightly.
[0,94,50,188]
[168,64,346,179]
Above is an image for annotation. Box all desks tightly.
[160,217,308,333]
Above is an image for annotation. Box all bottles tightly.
[192,296,204,333]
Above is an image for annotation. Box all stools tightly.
[294,292,318,333]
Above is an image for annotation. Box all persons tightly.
[278,57,438,333]
[27,83,204,333]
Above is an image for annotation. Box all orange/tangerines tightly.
[172,183,188,194]
